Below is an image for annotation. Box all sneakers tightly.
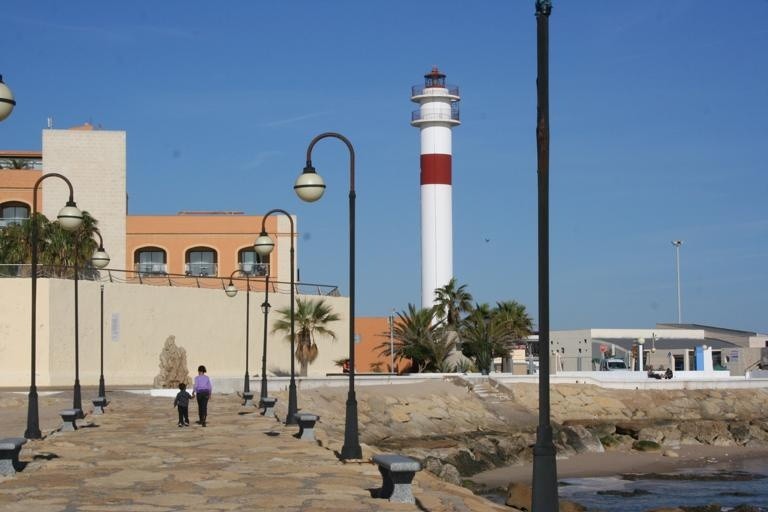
[178,415,207,429]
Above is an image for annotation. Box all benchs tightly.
[293,413,318,440]
[0,437,26,475]
[92,397,105,414]
[372,456,421,504]
[58,408,80,431]
[261,397,277,417]
[242,392,254,407]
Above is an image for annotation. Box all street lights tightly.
[25,172,84,439]
[73,227,111,419]
[293,131,363,458]
[224,261,253,393]
[254,208,300,427]
[671,240,683,324]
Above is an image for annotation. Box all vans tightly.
[599,358,629,371]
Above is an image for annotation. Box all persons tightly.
[342,359,350,373]
[191,365,212,427]
[664,367,674,379]
[647,364,661,379]
[174,382,195,428]
